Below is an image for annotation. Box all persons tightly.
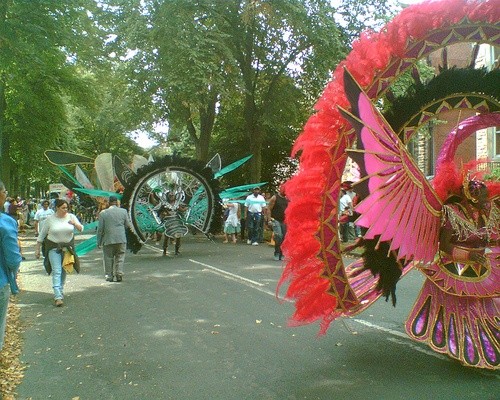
[33,199,55,259]
[258,191,276,245]
[69,195,107,225]
[154,190,190,255]
[217,196,250,244]
[339,180,363,244]
[267,185,289,261]
[243,186,268,246]
[96,195,131,283]
[34,200,85,307]
[0,181,23,355]
[404,180,499,370]
[4,192,61,230]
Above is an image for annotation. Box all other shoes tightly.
[252,242,259,246]
[163,252,168,256]
[223,240,228,244]
[279,255,285,260]
[55,300,63,307]
[175,251,180,255]
[116,275,122,281]
[247,239,252,244]
[233,239,237,244]
[273,255,279,260]
[105,277,113,282]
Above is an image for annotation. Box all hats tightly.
[253,187,260,190]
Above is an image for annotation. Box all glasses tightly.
[0,191,8,194]
